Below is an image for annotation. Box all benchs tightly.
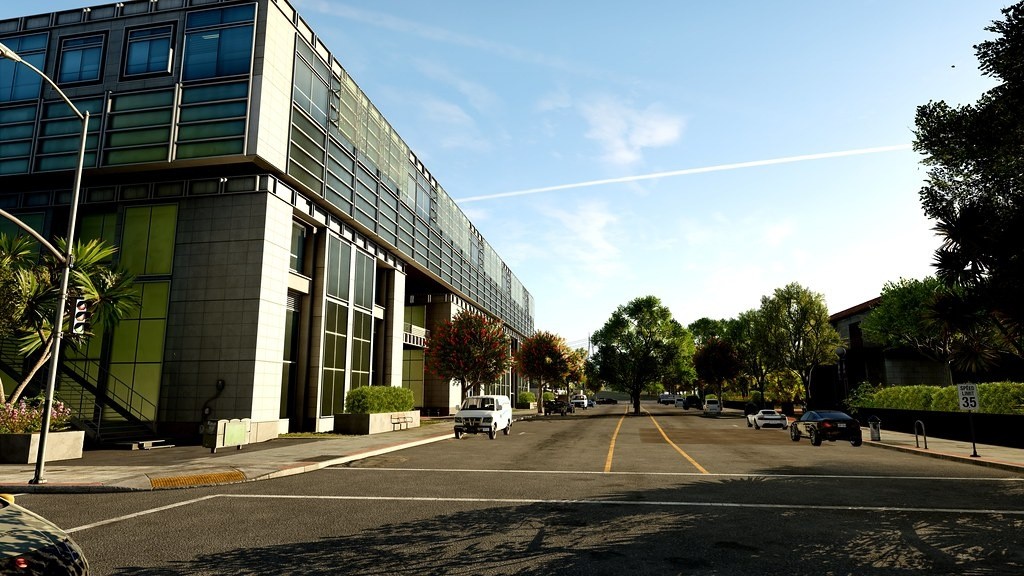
[391,413,413,431]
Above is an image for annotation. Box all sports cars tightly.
[746,409,789,431]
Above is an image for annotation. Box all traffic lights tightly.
[68,298,87,335]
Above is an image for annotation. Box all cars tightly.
[702,399,720,416]
[588,399,594,407]
[791,409,863,447]
[675,398,685,408]
[683,396,703,410]
[545,401,567,416]
[564,402,575,413]
[571,395,587,410]
[0,494,90,576]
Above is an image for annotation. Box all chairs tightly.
[468,405,477,408]
[489,400,501,410]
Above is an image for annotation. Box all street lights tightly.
[0,40,88,486]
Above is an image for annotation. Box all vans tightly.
[455,395,512,439]
